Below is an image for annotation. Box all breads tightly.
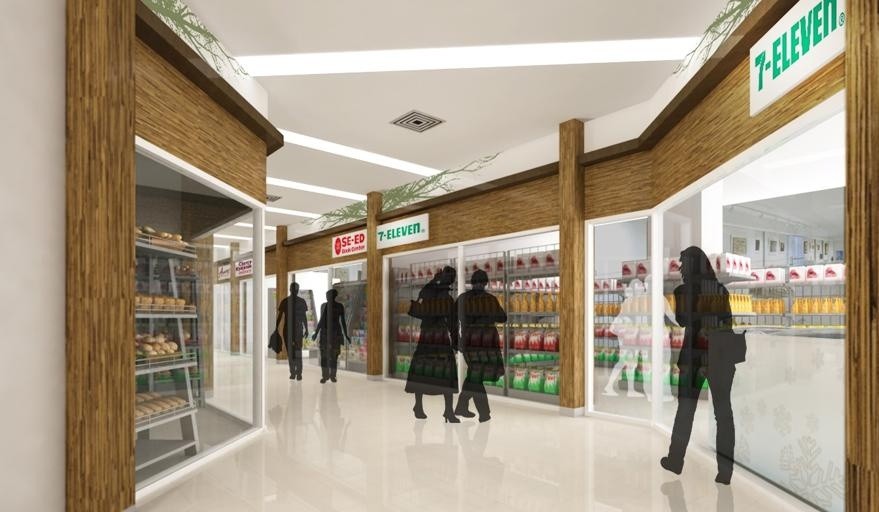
[134,225,187,250]
[182,329,191,339]
[135,293,186,311]
[135,390,187,418]
[135,332,177,357]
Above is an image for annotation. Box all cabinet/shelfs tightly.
[135,229,201,485]
[728,285,846,337]
[332,281,368,375]
[594,276,717,401]
[268,289,319,359]
[507,241,561,405]
[454,251,507,395]
[391,256,457,379]
